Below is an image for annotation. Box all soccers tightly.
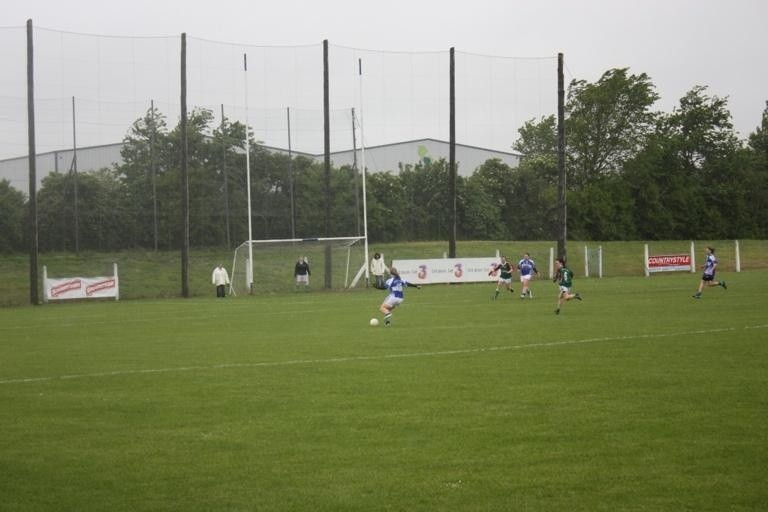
[370,319,379,326]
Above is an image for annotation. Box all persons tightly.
[513,252,541,300]
[211,262,230,297]
[370,253,389,289]
[293,255,311,294]
[691,245,728,300]
[487,255,514,302]
[551,258,582,316]
[373,268,422,327]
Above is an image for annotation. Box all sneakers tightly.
[383,312,392,327]
[491,289,583,315]
[692,280,727,300]
